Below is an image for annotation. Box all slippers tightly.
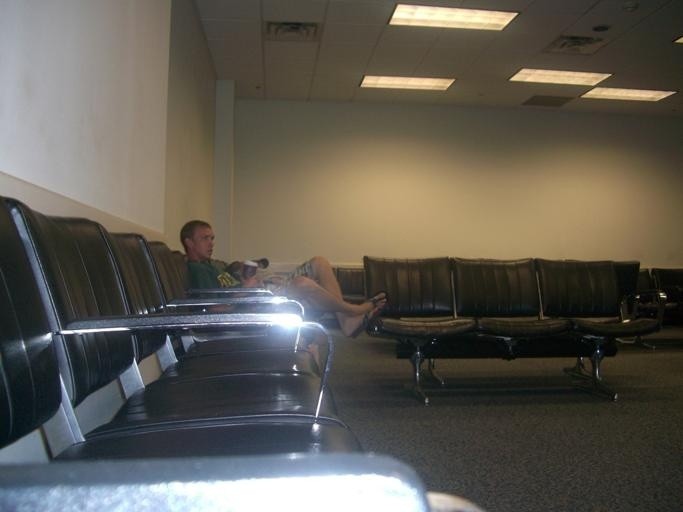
[347,294,387,340]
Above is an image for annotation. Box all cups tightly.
[242,260,257,280]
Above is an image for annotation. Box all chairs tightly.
[1,193,432,511]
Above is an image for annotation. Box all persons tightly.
[180,220,388,339]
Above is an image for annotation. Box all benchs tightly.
[330,256,683,404]
